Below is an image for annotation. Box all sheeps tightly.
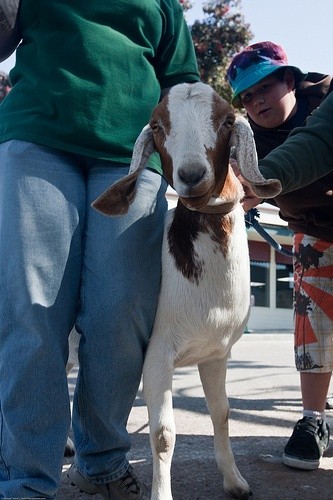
[65,81,283,500]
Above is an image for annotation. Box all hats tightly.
[227,41,309,109]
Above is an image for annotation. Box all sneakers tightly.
[282,416,330,470]
[67,462,151,500]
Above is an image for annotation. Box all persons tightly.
[225,40,332,469]
[0,1,201,500]
